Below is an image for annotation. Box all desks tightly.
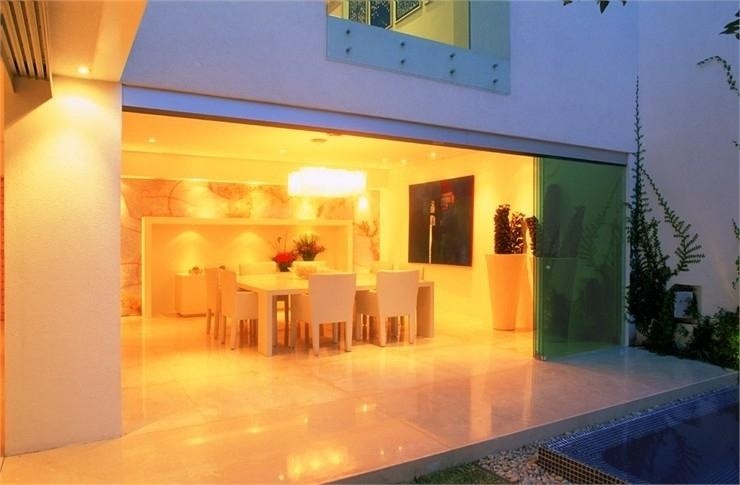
[235,270,435,353]
[177,269,216,318]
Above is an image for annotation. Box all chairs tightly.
[199,258,426,357]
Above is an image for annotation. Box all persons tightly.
[433,182,465,265]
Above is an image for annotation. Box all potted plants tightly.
[482,197,587,341]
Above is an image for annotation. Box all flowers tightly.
[271,234,326,267]
